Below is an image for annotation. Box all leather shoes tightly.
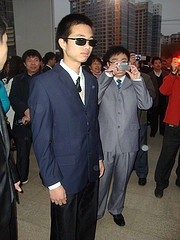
[109,212,125,226]
[154,186,164,198]
[138,177,146,185]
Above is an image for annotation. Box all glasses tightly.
[64,37,98,47]
[108,60,127,64]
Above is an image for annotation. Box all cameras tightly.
[117,63,129,71]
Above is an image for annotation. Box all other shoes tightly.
[21,177,28,184]
[150,129,158,137]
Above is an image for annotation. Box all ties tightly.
[76,77,82,92]
[117,79,122,89]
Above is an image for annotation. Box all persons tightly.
[0,15,60,240]
[28,13,105,240]
[95,46,153,227]
[85,51,180,197]
